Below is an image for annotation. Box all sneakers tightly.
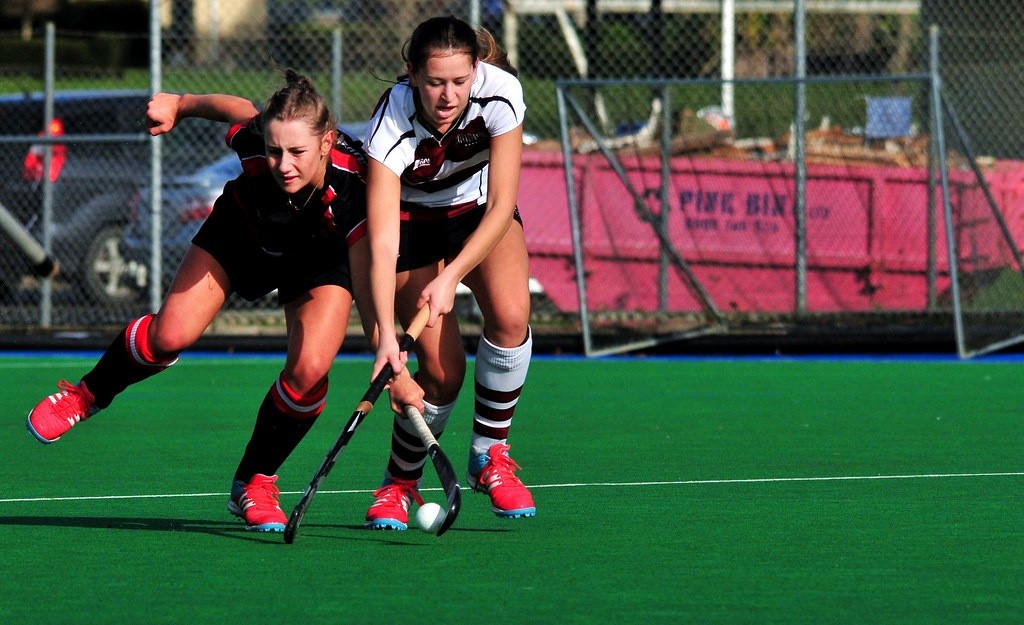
[25,379,101,445]
[466,439,536,519]
[365,477,424,531]
[227,473,288,532]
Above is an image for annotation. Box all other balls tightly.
[415,502,446,534]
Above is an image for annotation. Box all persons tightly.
[25,69,425,533]
[365,15,537,530]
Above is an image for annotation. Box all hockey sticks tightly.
[283,297,433,546]
[390,367,462,536]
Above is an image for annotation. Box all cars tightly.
[0,90,239,307]
[123,120,537,311]
